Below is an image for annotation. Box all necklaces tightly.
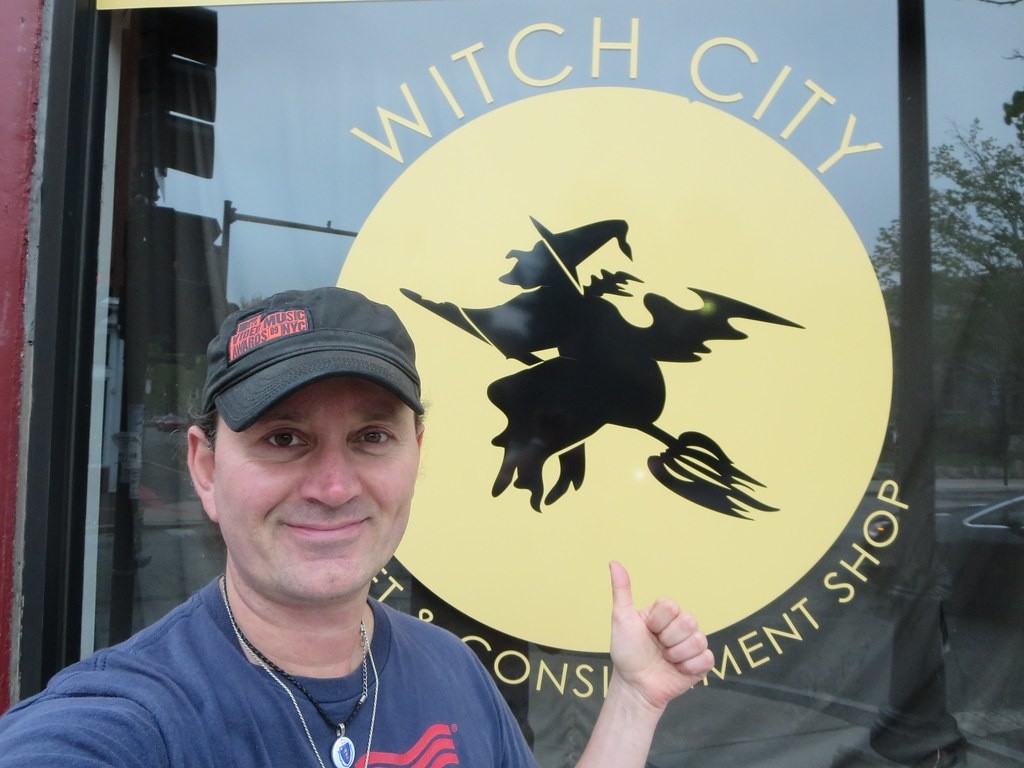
[222,586,379,768]
[224,577,369,767]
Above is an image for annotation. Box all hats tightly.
[202,285,424,433]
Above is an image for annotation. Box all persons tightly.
[1,287,713,768]
